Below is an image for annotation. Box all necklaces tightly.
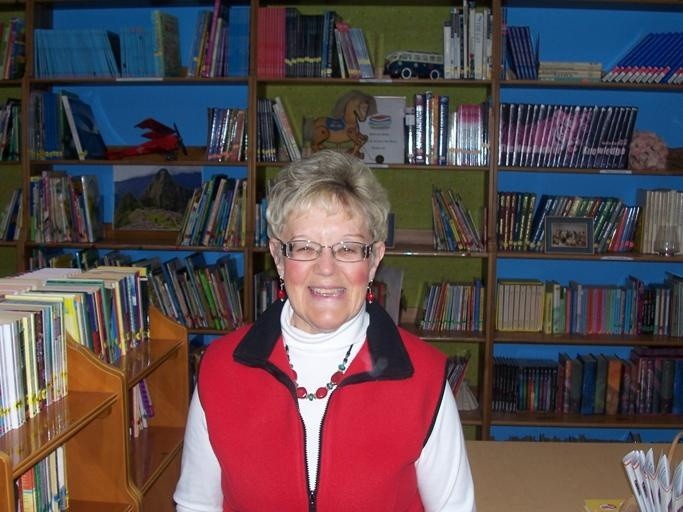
[274,322,361,402]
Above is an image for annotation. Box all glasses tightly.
[279,238,374,262]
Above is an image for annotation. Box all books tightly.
[2,2,250,330]
[492,7,682,416]
[0,267,155,439]
[10,443,71,512]
[257,2,494,413]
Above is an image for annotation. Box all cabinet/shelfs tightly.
[0,0,683,439]
[0,258,193,512]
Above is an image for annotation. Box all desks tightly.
[455,432,682,512]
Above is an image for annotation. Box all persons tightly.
[167,144,478,512]
